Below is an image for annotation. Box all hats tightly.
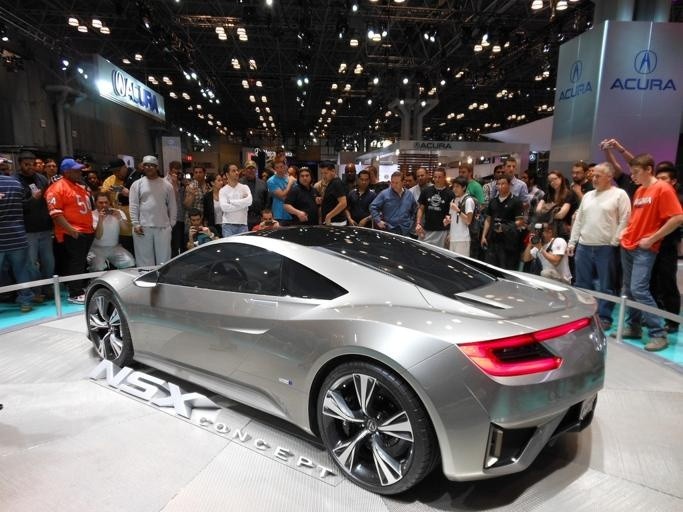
[106,155,160,172]
[244,160,256,167]
[59,158,86,172]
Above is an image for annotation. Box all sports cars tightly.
[84,225,607,496]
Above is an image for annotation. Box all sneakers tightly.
[601,322,668,351]
[67,294,85,304]
[21,294,46,312]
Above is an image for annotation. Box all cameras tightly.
[491,219,501,230]
[192,226,202,231]
[176,172,184,180]
[112,186,122,192]
[264,220,274,226]
[103,209,113,214]
[531,224,543,245]
[602,142,614,148]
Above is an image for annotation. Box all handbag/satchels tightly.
[502,221,524,250]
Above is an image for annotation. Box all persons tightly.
[600,139,642,205]
[343,156,631,330]
[609,153,683,351]
[1,151,347,313]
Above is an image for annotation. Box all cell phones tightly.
[28,183,39,191]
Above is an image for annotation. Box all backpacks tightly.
[459,195,481,240]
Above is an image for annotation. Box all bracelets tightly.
[619,147,624,153]
